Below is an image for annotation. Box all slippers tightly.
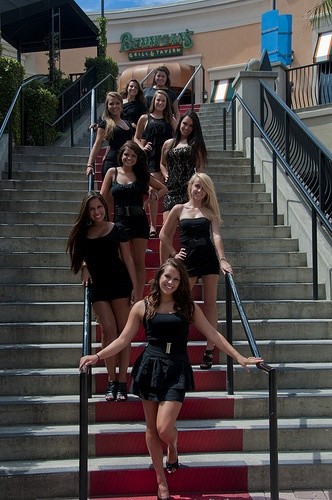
[149,231,157,238]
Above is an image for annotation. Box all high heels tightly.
[105,380,118,401]
[116,381,127,402]
[157,482,170,500]
[200,350,215,369]
[166,446,178,474]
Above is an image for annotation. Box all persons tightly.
[160,110,208,267]
[88,79,148,129]
[78,259,264,499]
[142,66,181,122]
[65,190,134,401]
[85,92,138,188]
[98,140,168,302]
[133,90,180,238]
[158,173,232,370]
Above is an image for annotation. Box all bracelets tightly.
[95,353,101,362]
[219,257,228,262]
